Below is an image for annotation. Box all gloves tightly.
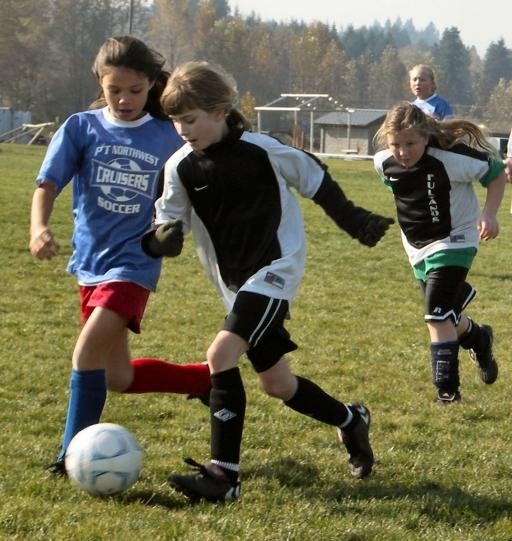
[141,220,183,259]
[355,212,394,246]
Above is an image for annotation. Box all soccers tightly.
[64,422,143,497]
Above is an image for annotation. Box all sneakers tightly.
[468,325,497,384]
[168,458,241,504]
[433,388,461,407]
[337,403,373,478]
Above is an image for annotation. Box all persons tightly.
[28,35,212,476]
[502,132,512,215]
[141,59,394,503]
[408,64,455,120]
[372,102,507,402]
